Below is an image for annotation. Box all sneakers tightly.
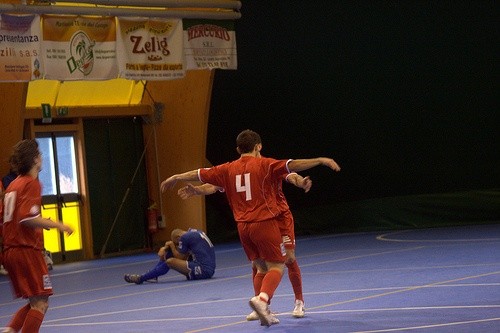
[292,300,305,317]
[125,273,142,284]
[247,310,259,320]
[259,316,279,326]
[249,296,271,327]
[148,277,158,283]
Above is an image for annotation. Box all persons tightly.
[124,228,216,285]
[177,152,311,320]
[0,139,74,333]
[160,129,341,327]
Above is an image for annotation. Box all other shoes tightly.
[0,268,8,275]
